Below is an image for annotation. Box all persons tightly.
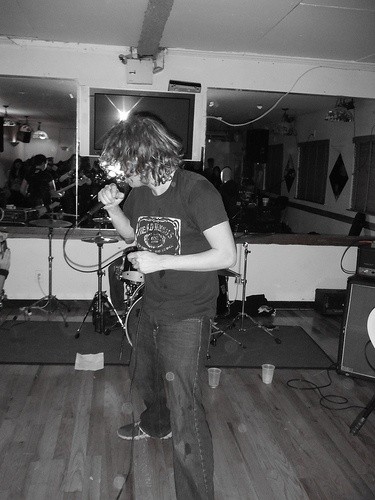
[10,153,240,228]
[98,116,238,500]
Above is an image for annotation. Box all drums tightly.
[125,282,144,347]
[119,245,145,286]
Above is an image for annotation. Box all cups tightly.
[207,368,221,387]
[262,197,269,206]
[262,363,275,384]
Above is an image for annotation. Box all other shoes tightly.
[116,420,173,440]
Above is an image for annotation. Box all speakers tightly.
[336,275,375,382]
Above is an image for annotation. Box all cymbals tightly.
[45,212,80,217]
[80,235,119,244]
[93,216,111,224]
[29,217,73,228]
[217,269,241,277]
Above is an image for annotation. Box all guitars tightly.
[49,175,92,209]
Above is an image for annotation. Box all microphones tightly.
[78,189,119,224]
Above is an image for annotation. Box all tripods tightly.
[75,243,126,340]
[13,226,71,327]
[217,238,283,345]
